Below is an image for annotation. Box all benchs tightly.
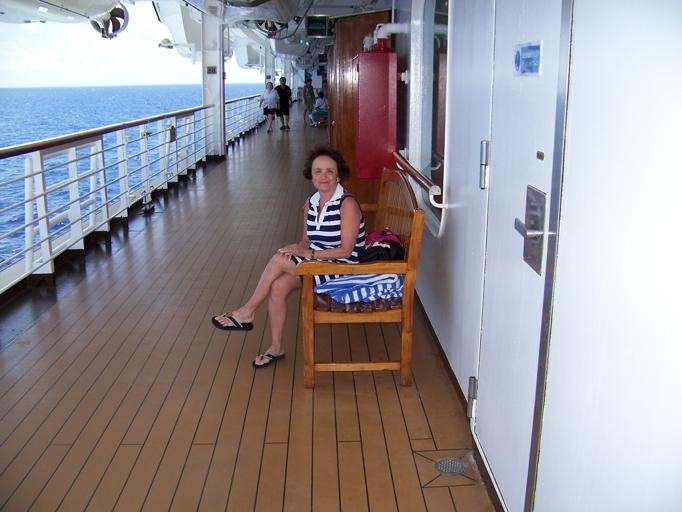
[292,168,426,390]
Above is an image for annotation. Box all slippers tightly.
[253,352,285,368]
[212,312,253,330]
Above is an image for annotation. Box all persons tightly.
[257,81,281,133]
[301,78,328,128]
[274,76,293,132]
[212,145,367,371]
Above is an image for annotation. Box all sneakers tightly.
[267,119,316,132]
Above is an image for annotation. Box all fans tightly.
[90,3,129,39]
